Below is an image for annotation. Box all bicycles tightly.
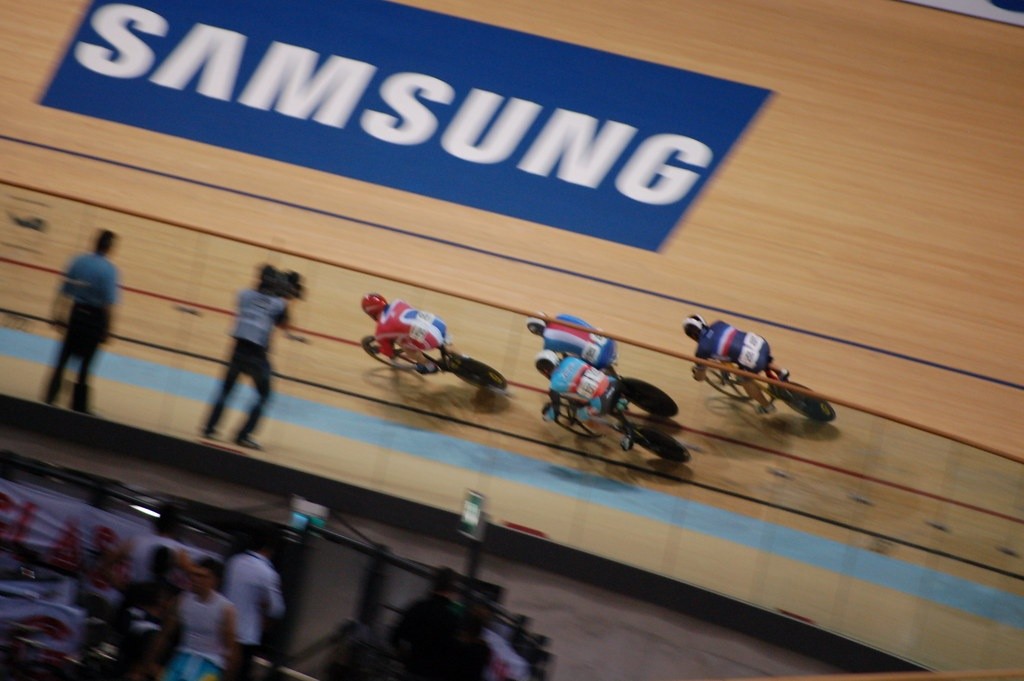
[690,355,838,424]
[361,335,513,393]
[558,348,682,418]
[542,397,693,464]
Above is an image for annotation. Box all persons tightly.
[43,226,120,416]
[100,524,540,681]
[201,262,290,452]
[533,350,633,450]
[361,293,455,374]
[684,315,792,416]
[526,310,618,371]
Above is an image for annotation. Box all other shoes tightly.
[203,429,218,441]
[425,361,438,374]
[778,367,789,381]
[621,435,632,450]
[755,403,775,415]
[237,438,261,449]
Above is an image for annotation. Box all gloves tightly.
[543,408,558,422]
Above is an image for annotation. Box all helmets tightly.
[682,314,706,341]
[527,311,549,335]
[360,293,387,316]
[534,350,560,378]
